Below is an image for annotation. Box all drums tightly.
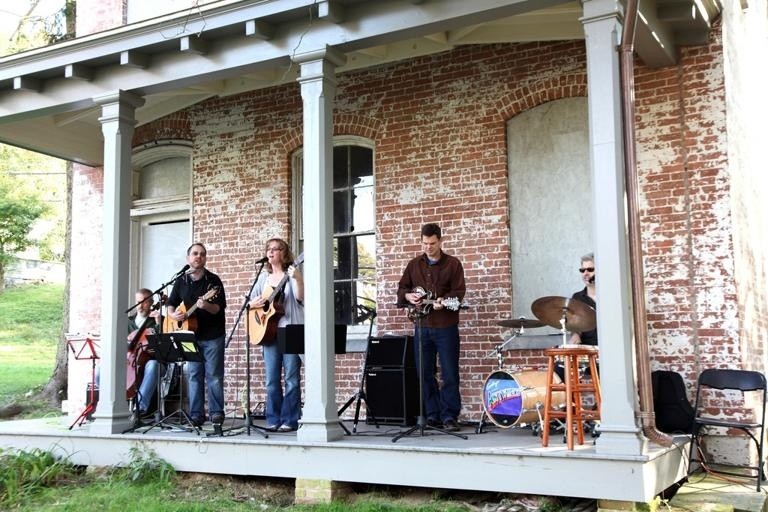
[481,368,567,429]
[554,343,599,367]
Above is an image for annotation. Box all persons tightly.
[94,289,166,412]
[552,253,598,404]
[395,222,466,429]
[246,238,303,431]
[167,241,227,428]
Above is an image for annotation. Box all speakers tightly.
[363,336,419,426]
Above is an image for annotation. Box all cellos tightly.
[126,292,167,400]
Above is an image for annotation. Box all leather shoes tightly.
[264,425,278,432]
[424,418,442,429]
[187,416,204,426]
[212,413,224,424]
[444,420,459,431]
[277,426,292,432]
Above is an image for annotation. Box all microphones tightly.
[149,301,165,308]
[255,257,270,264]
[178,264,191,274]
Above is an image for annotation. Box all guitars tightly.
[163,283,221,333]
[407,287,469,320]
[244,252,304,344]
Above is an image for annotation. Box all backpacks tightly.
[652,371,697,432]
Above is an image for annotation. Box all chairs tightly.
[651,370,709,462]
[686,369,766,492]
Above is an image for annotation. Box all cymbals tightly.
[497,318,544,328]
[530,296,596,333]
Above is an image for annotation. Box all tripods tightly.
[207,305,286,439]
[337,318,382,435]
[142,360,202,436]
[121,300,174,434]
[391,317,468,444]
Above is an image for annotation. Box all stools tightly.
[541,348,602,450]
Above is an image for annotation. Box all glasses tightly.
[579,267,594,272]
[192,252,205,257]
[266,247,284,251]
